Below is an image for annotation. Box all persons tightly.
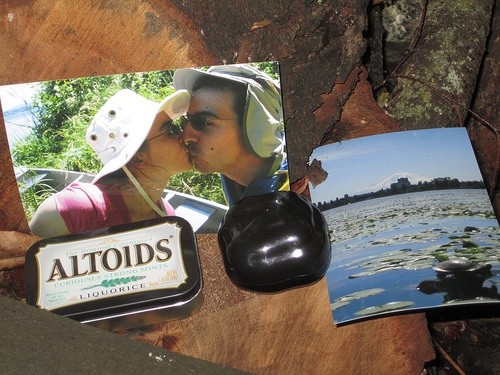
[172,63,290,209]
[28,89,195,241]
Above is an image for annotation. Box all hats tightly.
[173,65,284,160]
[86,86,190,184]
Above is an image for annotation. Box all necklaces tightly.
[124,182,164,192]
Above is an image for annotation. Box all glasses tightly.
[179,116,238,131]
[145,119,181,142]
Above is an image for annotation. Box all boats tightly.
[13,164,229,237]
